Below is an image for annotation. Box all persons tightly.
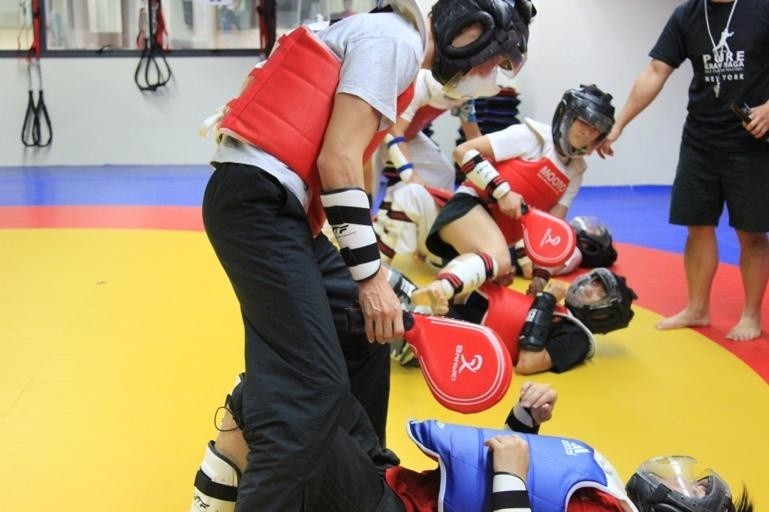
[586,0,769,342]
[373,65,501,194]
[192,371,751,512]
[203,0,537,511]
[408,83,615,316]
[374,183,617,290]
[380,261,639,377]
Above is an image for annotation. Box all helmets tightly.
[432,0,535,96]
[568,268,638,334]
[552,85,614,159]
[571,215,619,269]
[626,455,734,512]
